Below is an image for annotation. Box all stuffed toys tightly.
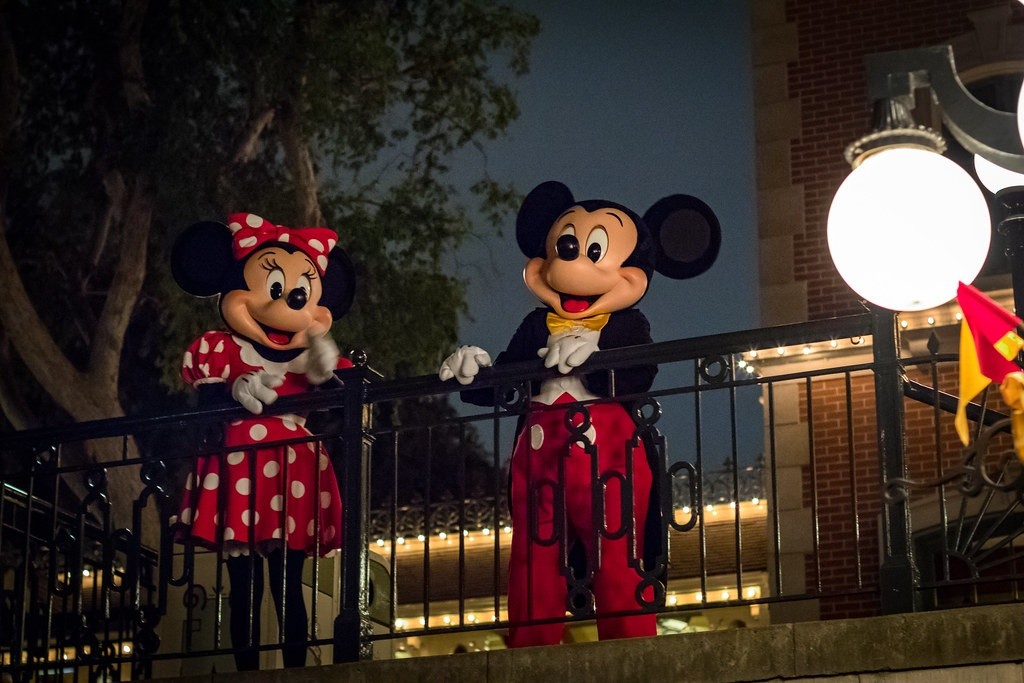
[440,180,722,648]
[170,213,354,672]
